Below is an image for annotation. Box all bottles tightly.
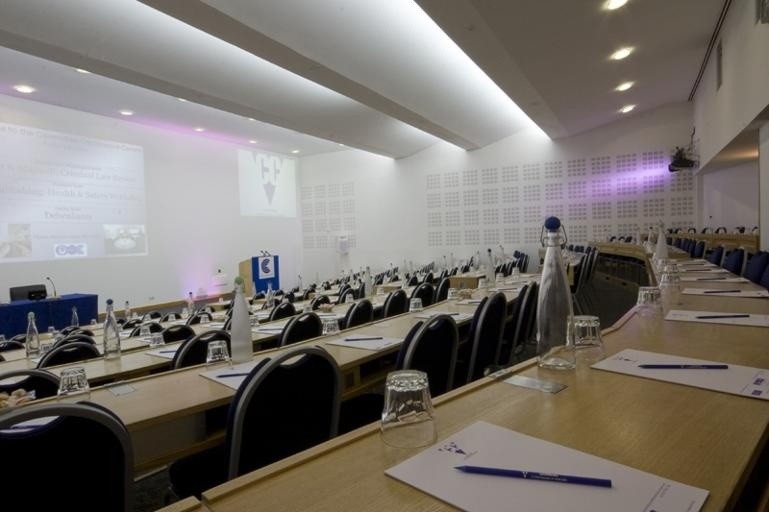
[102,299,121,360]
[188,292,195,317]
[123,301,131,322]
[26,311,40,360]
[708,216,714,238]
[536,215,580,372]
[654,225,668,260]
[364,266,373,298]
[71,305,80,329]
[484,249,495,283]
[647,226,656,246]
[267,279,275,311]
[230,276,254,362]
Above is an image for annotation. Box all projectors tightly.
[668,158,694,173]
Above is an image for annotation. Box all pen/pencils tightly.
[697,277,726,280]
[318,314,336,317]
[695,314,750,319]
[344,336,383,341]
[159,350,176,353]
[430,312,460,318]
[6,424,42,430]
[683,263,705,265]
[209,323,223,326]
[258,328,283,330]
[498,288,518,290]
[453,465,612,488]
[468,301,482,304]
[703,289,742,294]
[685,269,712,271]
[216,372,249,378]
[121,332,132,335]
[143,337,151,340]
[637,364,729,370]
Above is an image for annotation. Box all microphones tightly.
[46,277,56,296]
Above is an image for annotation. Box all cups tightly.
[511,267,519,279]
[409,298,423,312]
[303,304,312,312]
[574,315,605,362]
[447,288,458,299]
[479,279,488,288]
[151,332,165,346]
[40,344,50,355]
[208,341,230,362]
[637,286,663,315]
[140,326,150,337]
[494,272,505,286]
[322,319,340,336]
[59,367,89,395]
[168,314,175,321]
[656,272,682,304]
[249,315,259,326]
[381,370,436,449]
[346,294,353,303]
[200,314,209,324]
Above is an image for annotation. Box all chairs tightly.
[395,313,459,398]
[115,311,195,343]
[53,324,96,346]
[0,402,134,512]
[610,234,705,258]
[223,344,344,481]
[170,331,232,370]
[460,292,507,385]
[410,282,435,309]
[502,281,537,366]
[436,278,451,304]
[722,248,744,276]
[254,267,407,345]
[223,311,258,330]
[411,262,434,280]
[0,342,24,352]
[666,225,759,236]
[0,370,63,408]
[566,244,598,314]
[443,257,474,275]
[708,247,724,267]
[38,342,99,368]
[495,250,530,279]
[743,252,769,283]
[186,311,213,326]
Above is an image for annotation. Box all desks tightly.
[207,251,398,312]
[0,271,546,497]
[538,247,588,288]
[565,240,691,301]
[0,304,263,361]
[639,232,759,254]
[0,284,438,386]
[0,296,339,375]
[157,257,765,511]
[23,313,192,347]
[449,256,518,289]
[373,260,466,295]
